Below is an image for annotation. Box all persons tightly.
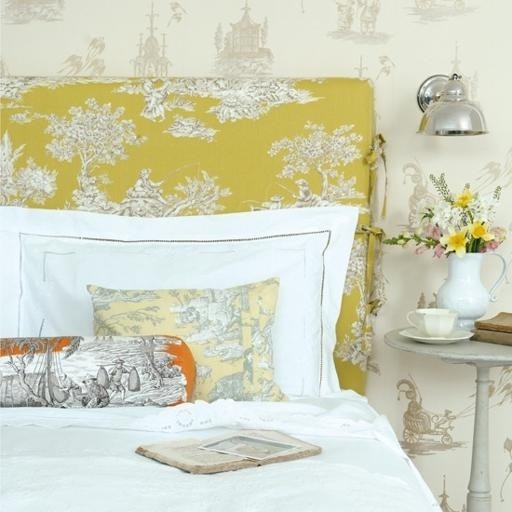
[292,179,321,202]
[134,168,167,204]
[107,358,130,403]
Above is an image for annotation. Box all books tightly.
[134,428,322,475]
[469,312,512,346]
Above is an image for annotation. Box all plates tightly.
[400,329,475,345]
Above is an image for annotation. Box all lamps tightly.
[409,68,492,139]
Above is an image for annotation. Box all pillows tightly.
[1,334,197,408]
[0,200,359,394]
[81,280,290,402]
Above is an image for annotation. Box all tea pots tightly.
[435,253,507,331]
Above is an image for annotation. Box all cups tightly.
[407,308,459,337]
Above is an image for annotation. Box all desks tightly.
[387,328,512,512]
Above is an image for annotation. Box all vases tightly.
[434,249,509,324]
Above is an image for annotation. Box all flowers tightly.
[380,163,510,258]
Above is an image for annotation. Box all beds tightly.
[0,72,448,512]
